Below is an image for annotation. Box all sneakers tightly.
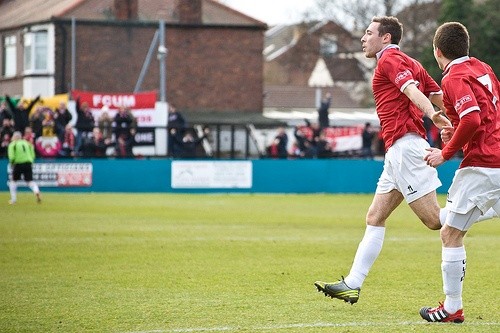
[314,275,360,305]
[8,200,16,205]
[420,301,464,323]
[36,192,41,203]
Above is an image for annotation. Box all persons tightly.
[8,131,41,205]
[314,16,500,306]
[419,22,500,323]
[0,93,466,158]
